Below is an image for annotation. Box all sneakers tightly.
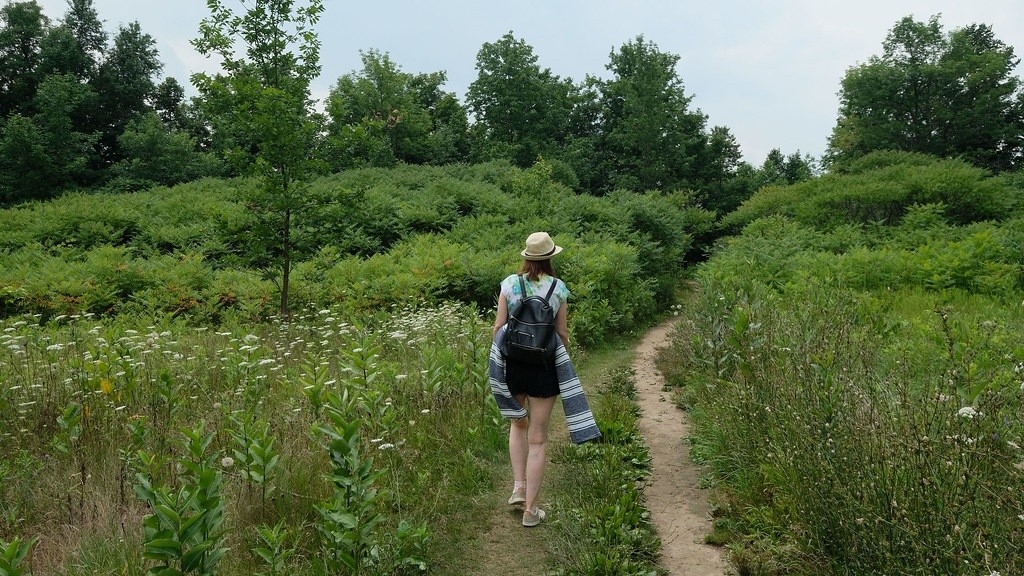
[523,506,546,526]
[508,487,526,504]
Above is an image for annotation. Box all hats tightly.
[521,232,563,261]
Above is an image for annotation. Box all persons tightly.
[494,233,571,526]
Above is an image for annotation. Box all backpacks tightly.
[503,274,558,365]
